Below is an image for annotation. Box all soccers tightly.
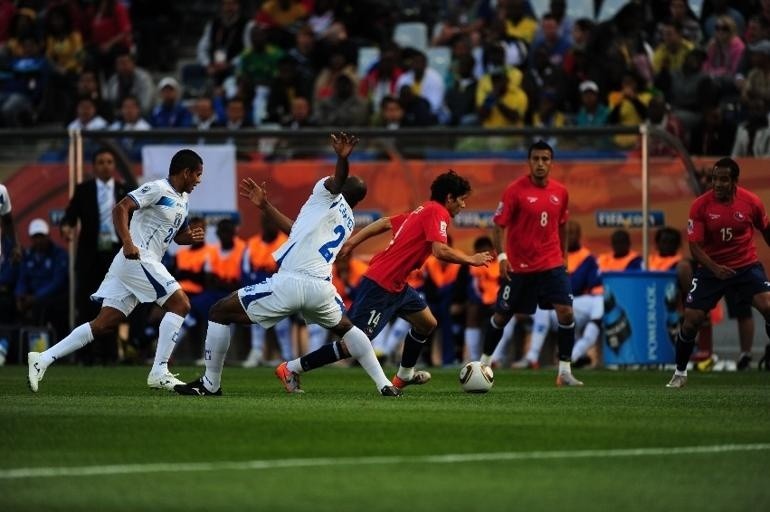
[458,360,494,393]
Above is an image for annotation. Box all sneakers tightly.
[381,384,403,397]
[173,376,224,397]
[274,360,307,396]
[712,359,726,373]
[725,359,736,375]
[555,372,584,388]
[665,372,689,389]
[509,356,541,371]
[737,348,755,372]
[146,369,188,392]
[26,350,48,394]
[392,369,432,389]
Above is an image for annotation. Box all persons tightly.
[2,2,769,157]
[2,133,769,395]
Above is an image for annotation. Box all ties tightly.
[95,187,117,252]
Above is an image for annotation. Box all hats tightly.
[577,79,600,95]
[26,217,50,240]
[156,76,180,93]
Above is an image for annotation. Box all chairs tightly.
[423,46,451,80]
[358,46,381,79]
[393,23,429,54]
